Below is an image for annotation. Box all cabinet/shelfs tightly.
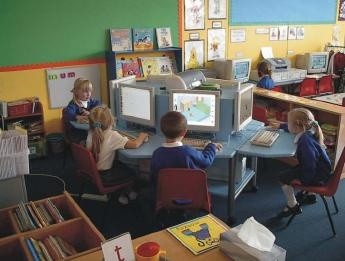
[0,99,46,159]
[252,86,345,180]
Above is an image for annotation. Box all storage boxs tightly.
[0,191,106,261]
[0,149,30,178]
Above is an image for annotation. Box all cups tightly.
[135,242,167,261]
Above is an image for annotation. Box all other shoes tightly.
[128,191,138,200]
[118,196,128,204]
[279,202,300,217]
[294,190,316,203]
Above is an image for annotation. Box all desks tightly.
[63,214,233,261]
[302,92,345,107]
[68,114,298,227]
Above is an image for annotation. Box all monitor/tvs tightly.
[168,88,221,135]
[234,86,253,136]
[295,51,330,75]
[118,82,155,132]
[213,59,252,83]
[108,73,136,117]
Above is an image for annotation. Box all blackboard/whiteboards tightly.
[45,64,102,109]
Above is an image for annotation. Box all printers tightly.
[164,69,206,93]
[262,56,291,73]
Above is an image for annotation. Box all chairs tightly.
[63,107,72,141]
[284,147,345,236]
[71,142,142,225]
[253,105,267,125]
[155,169,211,221]
[299,75,334,97]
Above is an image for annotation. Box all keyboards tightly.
[116,129,136,141]
[249,129,279,147]
[180,137,212,151]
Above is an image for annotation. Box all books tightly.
[111,28,132,51]
[156,28,172,49]
[8,119,43,132]
[133,27,153,50]
[13,200,65,232]
[166,215,230,256]
[116,56,177,79]
[26,235,77,261]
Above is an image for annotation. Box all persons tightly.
[64,77,101,144]
[86,104,150,205]
[256,61,275,90]
[151,110,223,228]
[269,108,332,217]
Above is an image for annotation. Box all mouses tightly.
[143,136,148,142]
